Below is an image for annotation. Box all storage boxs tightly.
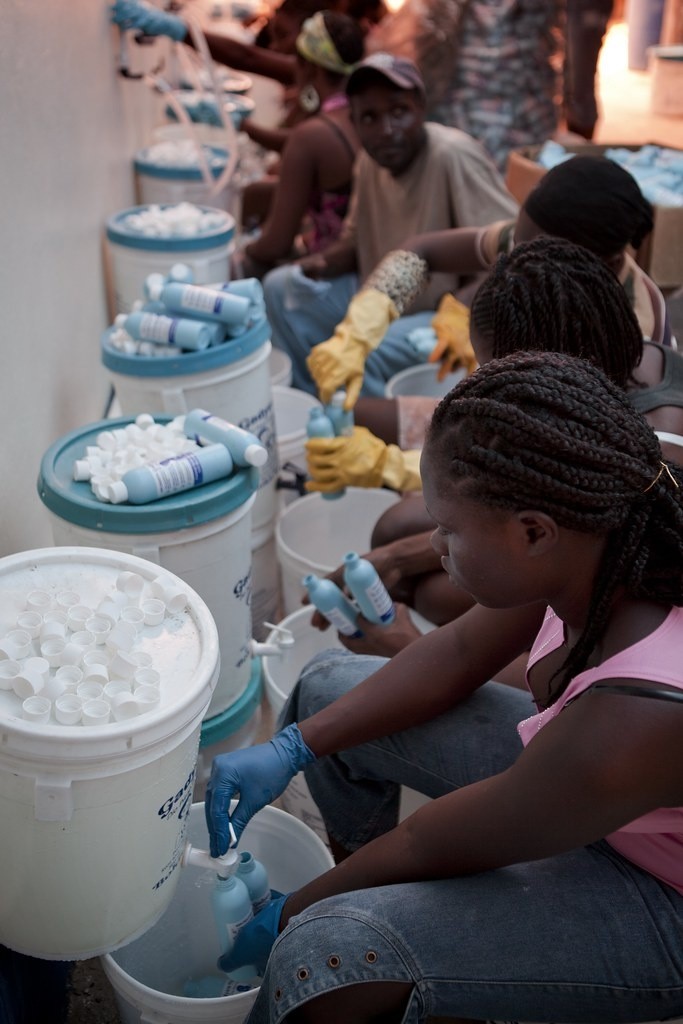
[505,141,682,298]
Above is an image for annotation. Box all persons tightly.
[205,350,683,1024]
[107,0,683,692]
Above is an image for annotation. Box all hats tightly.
[343,51,426,107]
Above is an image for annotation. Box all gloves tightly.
[217,891,294,976]
[428,293,478,383]
[205,725,316,858]
[306,287,402,412]
[305,426,423,496]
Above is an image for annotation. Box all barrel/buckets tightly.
[103,200,242,305]
[36,348,478,848]
[132,64,257,219]
[100,798,340,1024]
[0,547,218,960]
[100,311,280,530]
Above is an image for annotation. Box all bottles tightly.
[108,443,233,504]
[343,551,395,626]
[183,409,267,466]
[237,851,272,916]
[309,407,347,500]
[211,874,259,981]
[114,262,267,350]
[323,393,355,439]
[303,575,365,640]
